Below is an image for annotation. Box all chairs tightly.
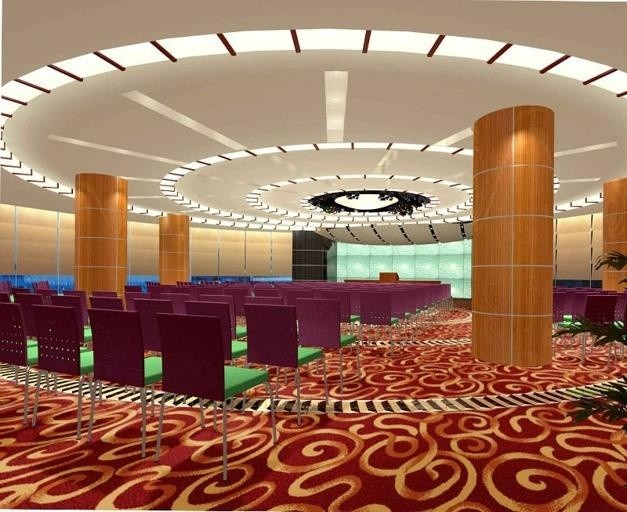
[1,281,456,482]
[552,284,626,367]
